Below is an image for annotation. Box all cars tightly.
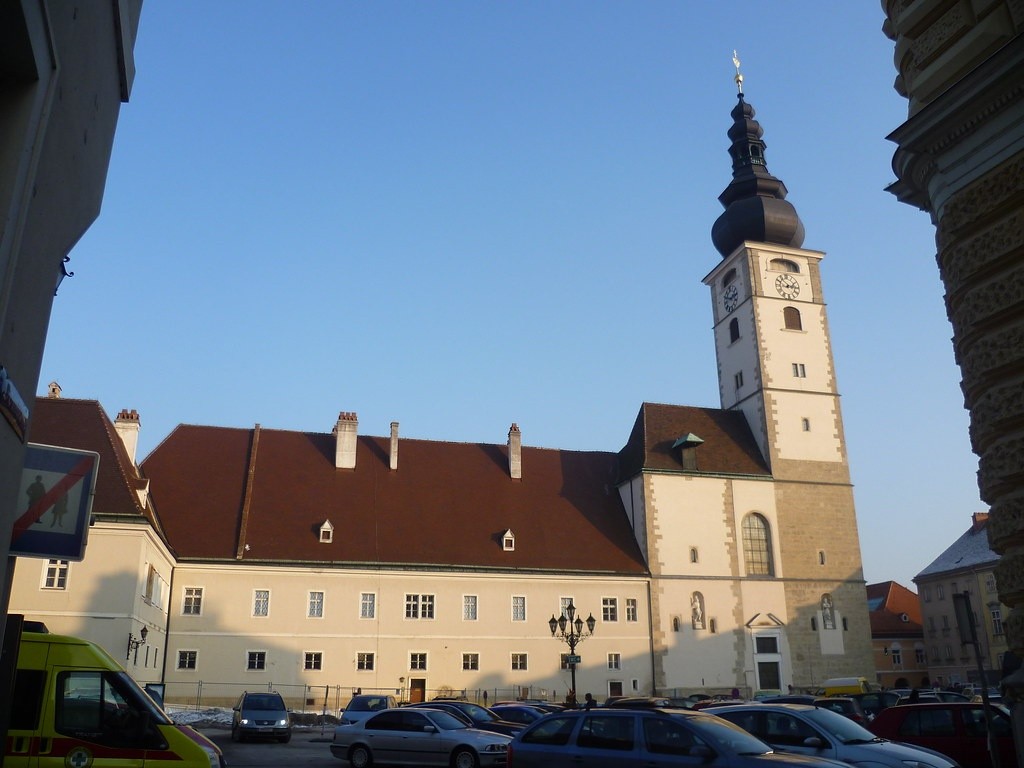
[865,702,1019,768]
[328,708,514,768]
[698,703,960,768]
[487,696,680,725]
[747,676,1002,727]
[338,694,399,726]
[526,698,566,711]
[400,700,530,737]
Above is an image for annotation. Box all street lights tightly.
[549,601,596,703]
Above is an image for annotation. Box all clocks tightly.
[775,274,801,300]
[724,285,738,313]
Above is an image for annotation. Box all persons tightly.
[353,688,361,697]
[823,598,833,628]
[456,691,468,701]
[692,597,701,621]
[585,693,597,708]
[905,690,920,705]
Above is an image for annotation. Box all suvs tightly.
[231,691,293,744]
[507,705,856,768]
[431,694,469,702]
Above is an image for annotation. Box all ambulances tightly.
[2,630,227,767]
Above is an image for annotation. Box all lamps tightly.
[126,626,150,660]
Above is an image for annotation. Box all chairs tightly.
[650,724,698,749]
[777,717,799,740]
